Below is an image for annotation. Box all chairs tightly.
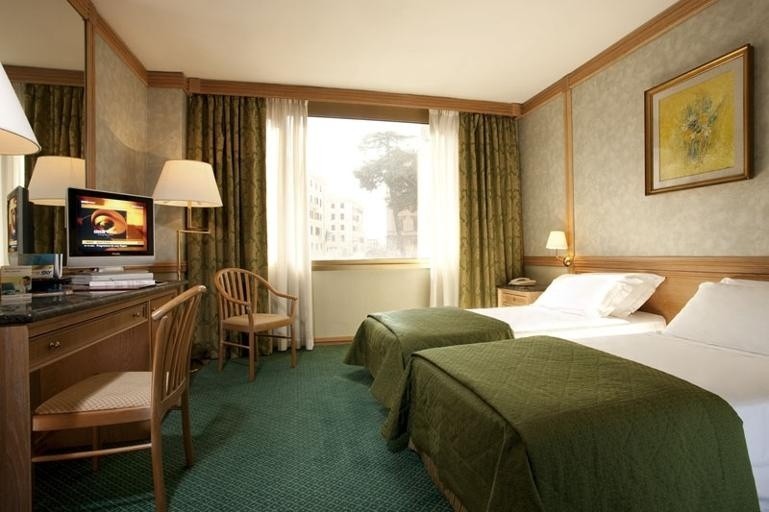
[213,267,300,382]
[28,284,208,511]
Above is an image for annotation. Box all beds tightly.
[382,278,769,510]
[343,271,665,410]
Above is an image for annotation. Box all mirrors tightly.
[0,0,89,266]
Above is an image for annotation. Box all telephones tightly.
[508,278,536,286]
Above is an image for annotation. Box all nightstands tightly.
[495,284,547,308]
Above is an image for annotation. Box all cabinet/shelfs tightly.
[27,289,182,373]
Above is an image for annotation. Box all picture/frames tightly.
[642,41,755,197]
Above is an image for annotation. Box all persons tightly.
[20,274,31,293]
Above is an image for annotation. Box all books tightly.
[69,272,155,283]
[72,280,156,291]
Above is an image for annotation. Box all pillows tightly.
[532,273,667,320]
[662,276,769,357]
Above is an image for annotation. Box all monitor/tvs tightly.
[7,186,54,265]
[65,186,156,280]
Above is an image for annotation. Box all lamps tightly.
[150,159,225,279]
[24,153,87,251]
[545,230,568,262]
